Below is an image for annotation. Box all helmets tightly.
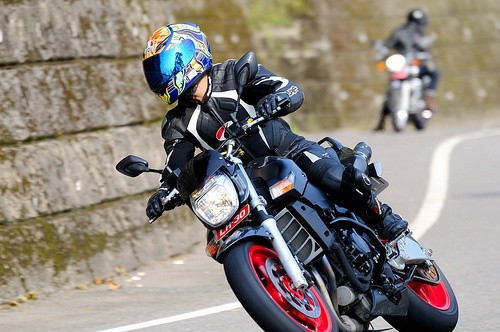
[407,9,429,27]
[143,23,213,105]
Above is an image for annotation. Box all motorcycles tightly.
[113,49,461,332]
[374,49,434,131]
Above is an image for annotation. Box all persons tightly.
[374,9,438,132]
[143,20,408,244]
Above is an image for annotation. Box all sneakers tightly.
[371,205,408,240]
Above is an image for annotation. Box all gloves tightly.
[145,190,167,219]
[257,93,281,128]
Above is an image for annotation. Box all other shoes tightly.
[424,92,437,113]
[374,119,386,131]
